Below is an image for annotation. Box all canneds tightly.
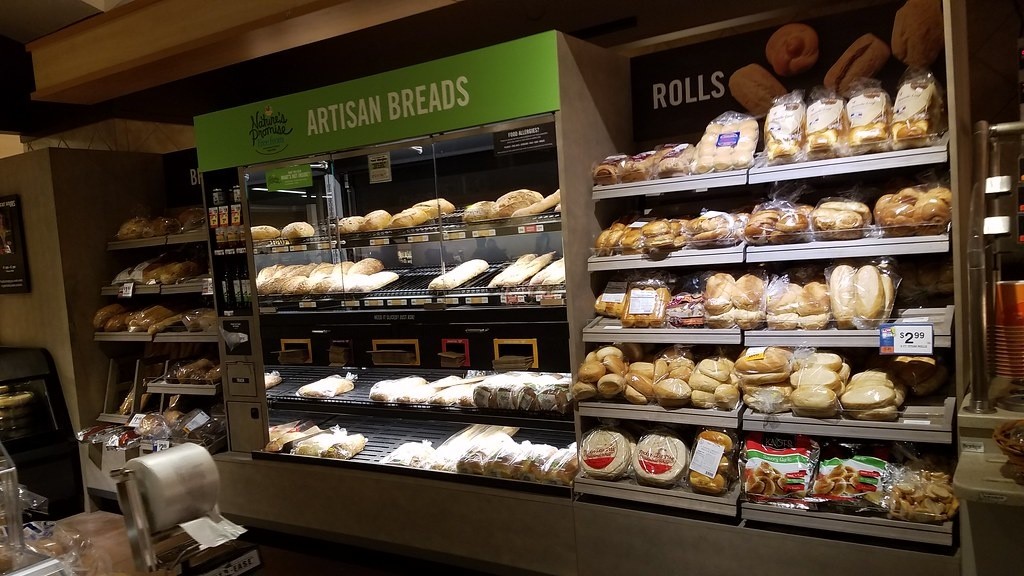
[212,185,241,206]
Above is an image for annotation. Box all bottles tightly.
[220,265,251,311]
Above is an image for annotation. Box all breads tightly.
[593,76,945,186]
[765,22,819,76]
[594,183,953,257]
[572,339,944,420]
[92,208,227,449]
[821,32,891,99]
[580,425,960,524]
[593,252,955,331]
[247,186,581,483]
[891,0,945,71]
[727,63,787,116]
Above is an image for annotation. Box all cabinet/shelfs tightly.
[92,0,1024,576]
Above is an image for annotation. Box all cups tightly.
[992,279,1024,380]
